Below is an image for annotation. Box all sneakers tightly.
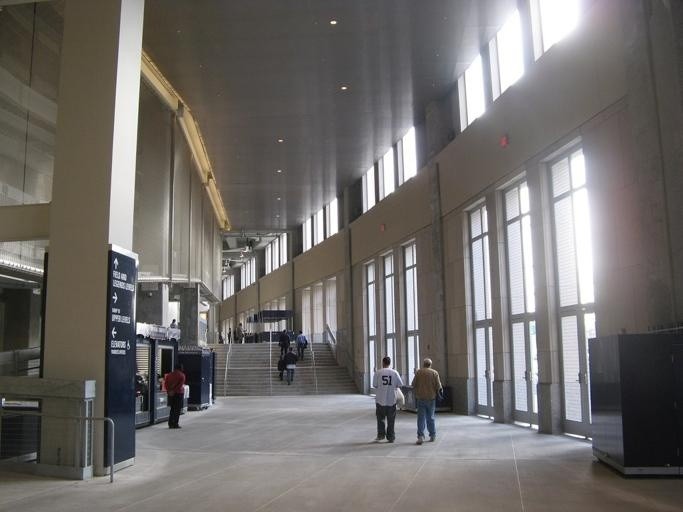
[376,436,395,442]
[416,436,436,444]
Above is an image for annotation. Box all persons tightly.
[278,356,285,380]
[237,324,247,343]
[170,319,177,328]
[372,357,404,442]
[227,327,232,344]
[135,370,169,410]
[285,347,298,385]
[296,331,306,360]
[279,330,290,355]
[164,362,186,429]
[411,357,442,444]
